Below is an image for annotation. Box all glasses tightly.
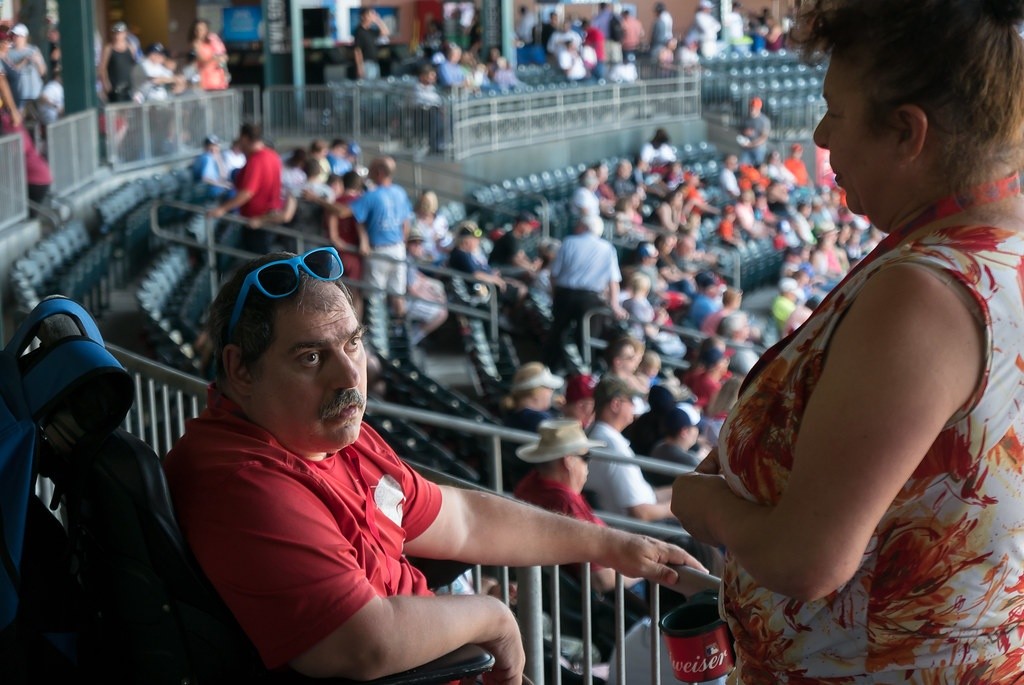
[228,246,345,345]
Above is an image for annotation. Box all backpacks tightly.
[0,295,264,685]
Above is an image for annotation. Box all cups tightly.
[659,599,736,683]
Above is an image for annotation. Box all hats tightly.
[639,242,660,260]
[515,418,609,463]
[518,208,542,229]
[701,348,737,367]
[203,133,222,147]
[663,400,701,426]
[150,41,173,59]
[816,221,840,238]
[9,23,29,37]
[110,22,128,32]
[407,227,427,244]
[750,96,762,110]
[697,1,716,8]
[512,369,566,389]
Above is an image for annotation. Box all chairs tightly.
[1,47,832,685]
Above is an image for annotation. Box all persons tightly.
[0,19,234,217]
[345,0,797,155]
[192,96,888,685]
[670,0,1024,685]
[163,251,711,685]
